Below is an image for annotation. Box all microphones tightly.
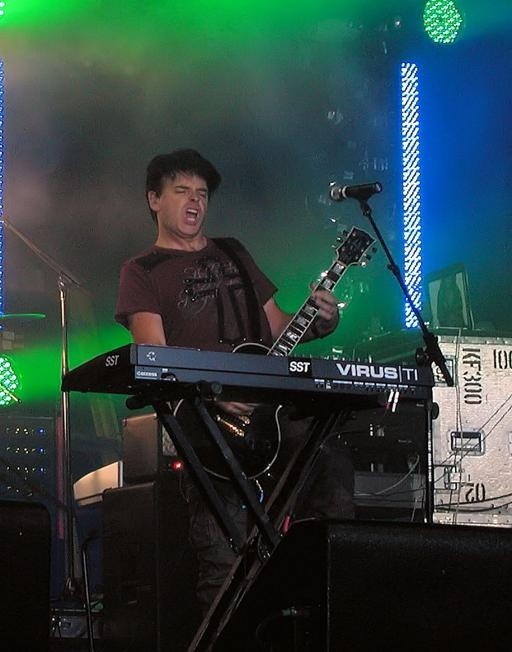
[329,181,383,202]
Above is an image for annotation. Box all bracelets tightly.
[310,322,325,339]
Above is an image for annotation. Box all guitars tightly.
[172,225,379,478]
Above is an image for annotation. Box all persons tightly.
[111,148,339,650]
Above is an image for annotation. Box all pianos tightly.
[62,343,435,409]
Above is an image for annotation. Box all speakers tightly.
[0,500,52,652]
[281,517,512,652]
[101,481,203,652]
